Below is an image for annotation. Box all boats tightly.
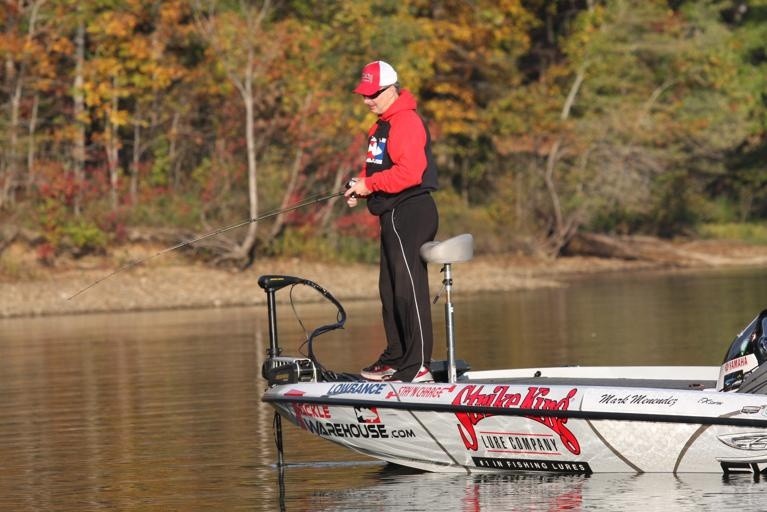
[256,232,767,477]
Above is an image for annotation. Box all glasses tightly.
[367,85,391,101]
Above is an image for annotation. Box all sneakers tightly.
[360,361,396,381]
[379,365,435,384]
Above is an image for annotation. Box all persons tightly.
[340,57,439,386]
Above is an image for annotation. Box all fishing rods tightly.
[65,181,358,302]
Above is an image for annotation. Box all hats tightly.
[351,60,398,97]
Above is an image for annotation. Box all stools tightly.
[420,233,474,383]
